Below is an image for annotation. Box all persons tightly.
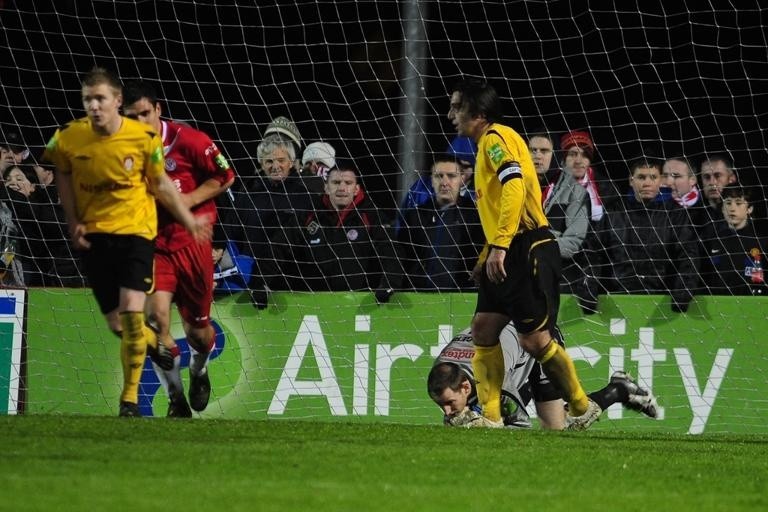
[447,75,603,433]
[118,78,235,418]
[428,313,660,431]
[1,118,765,295]
[45,70,215,418]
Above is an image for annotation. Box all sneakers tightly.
[609,368,665,421]
[166,399,193,419]
[144,320,175,370]
[187,366,211,411]
[558,396,604,432]
[117,400,141,417]
[458,416,506,428]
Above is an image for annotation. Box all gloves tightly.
[249,287,269,310]
[672,293,692,313]
[373,284,395,307]
[581,293,599,314]
[451,407,478,428]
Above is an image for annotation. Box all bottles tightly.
[751,257,764,297]
[0,239,17,278]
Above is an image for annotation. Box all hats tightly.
[262,114,302,150]
[445,135,475,165]
[301,141,337,168]
[558,130,594,151]
